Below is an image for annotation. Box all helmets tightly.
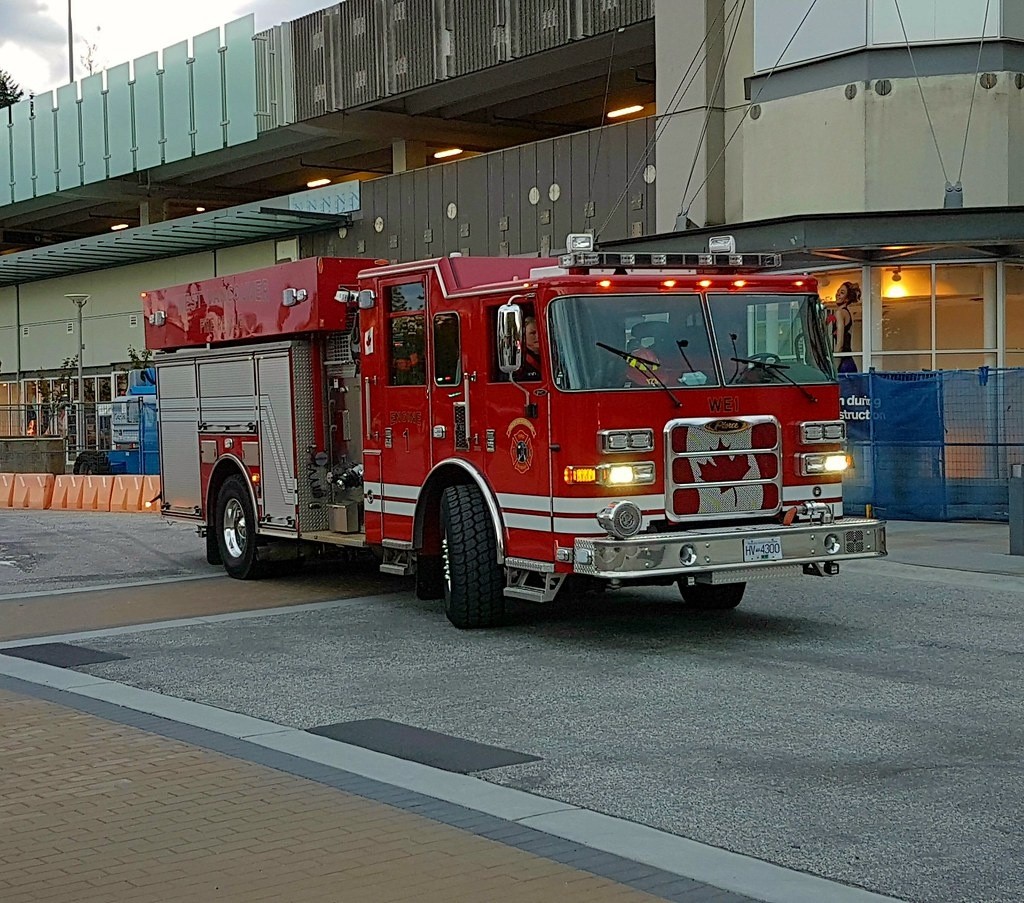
[625,348,669,386]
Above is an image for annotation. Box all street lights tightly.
[66,294,90,458]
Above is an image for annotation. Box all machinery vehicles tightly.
[73,369,161,475]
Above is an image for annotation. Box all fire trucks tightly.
[140,233,888,631]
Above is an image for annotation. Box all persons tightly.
[832,281,861,373]
[522,317,542,380]
